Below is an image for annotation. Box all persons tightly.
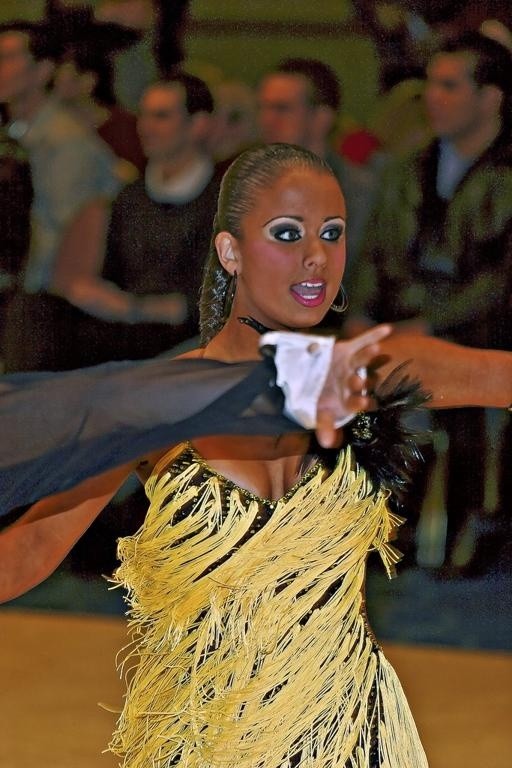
[1,141,512,768]
[1,324,393,516]
[1,19,512,582]
